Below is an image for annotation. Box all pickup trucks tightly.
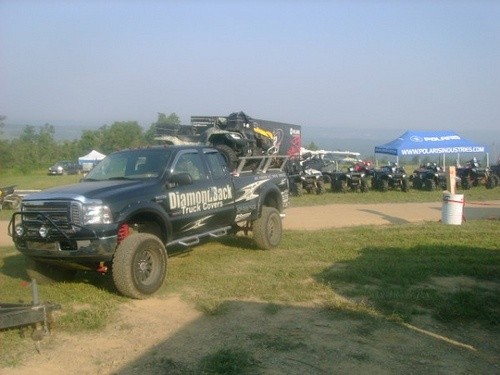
[6,142,290,300]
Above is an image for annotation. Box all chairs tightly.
[176,159,200,182]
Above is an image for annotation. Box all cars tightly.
[48,160,77,175]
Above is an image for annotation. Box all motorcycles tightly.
[285,151,500,197]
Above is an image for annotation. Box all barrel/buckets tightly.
[442,193,464,226]
